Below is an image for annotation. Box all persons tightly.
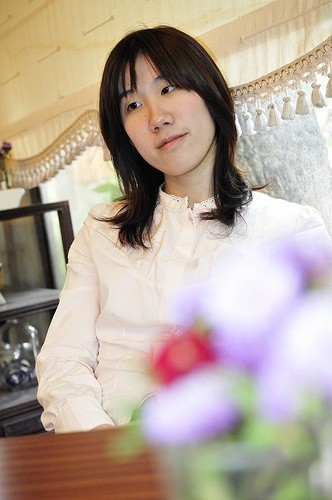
[35,24,332,437]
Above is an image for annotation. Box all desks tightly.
[0,424,171,499]
